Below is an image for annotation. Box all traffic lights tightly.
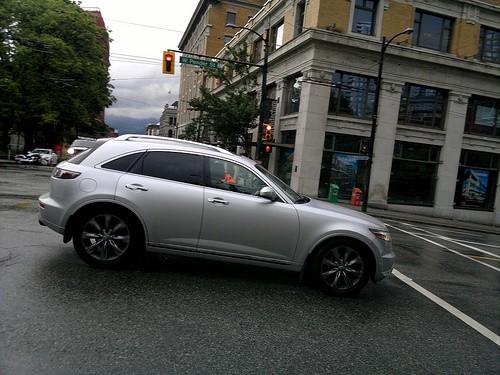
[162,51,175,74]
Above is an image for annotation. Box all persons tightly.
[211,163,230,190]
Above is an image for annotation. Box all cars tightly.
[25,148,58,165]
[67,136,97,159]
[37,132,397,298]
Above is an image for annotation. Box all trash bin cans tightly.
[351,188,362,205]
[329,184,339,201]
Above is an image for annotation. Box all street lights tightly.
[224,22,270,161]
[361,27,413,212]
